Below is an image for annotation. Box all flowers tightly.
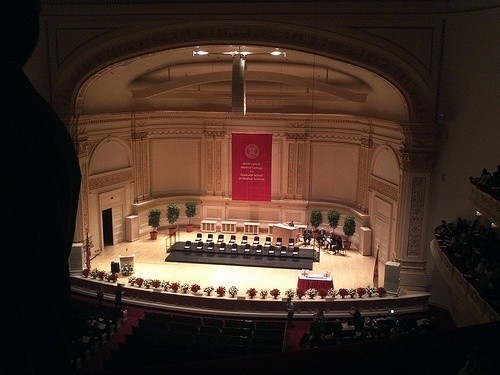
[80,268,387,298]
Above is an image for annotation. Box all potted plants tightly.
[311,208,357,250]
[149,201,197,240]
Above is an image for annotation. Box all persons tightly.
[285,297,294,328]
[434,216,500,288]
[288,220,294,227]
[0,0,82,375]
[303,228,343,255]
[97,286,104,305]
[469,165,500,188]
[298,310,440,349]
[114,284,123,308]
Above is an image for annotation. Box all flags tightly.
[373,248,379,289]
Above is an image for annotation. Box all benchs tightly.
[43,301,439,370]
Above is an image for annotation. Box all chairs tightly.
[184,233,300,258]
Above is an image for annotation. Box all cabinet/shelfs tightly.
[268,223,276,234]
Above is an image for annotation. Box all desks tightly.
[221,221,237,233]
[244,222,260,235]
[200,221,218,232]
[297,275,334,297]
